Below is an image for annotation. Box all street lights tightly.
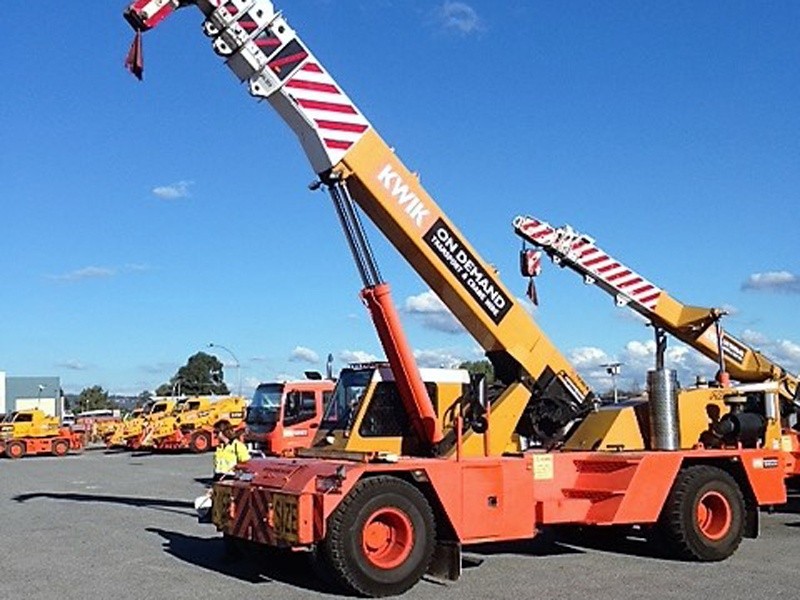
[600,362,625,404]
[205,342,243,397]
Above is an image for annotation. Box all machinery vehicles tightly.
[120,1,791,600]
[510,216,800,492]
[0,352,340,458]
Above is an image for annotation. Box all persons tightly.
[84,419,98,443]
[213,419,252,544]
